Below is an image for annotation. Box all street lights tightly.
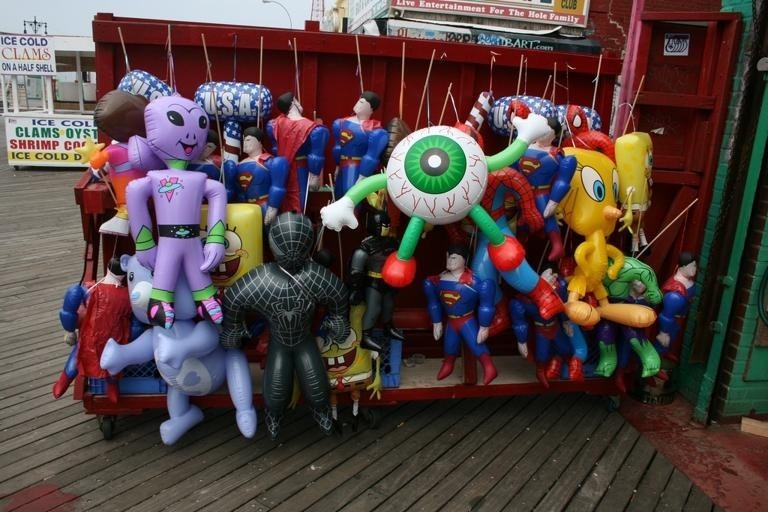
[22,15,49,35]
[263,0,293,29]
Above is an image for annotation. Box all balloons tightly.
[52,70,695,446]
